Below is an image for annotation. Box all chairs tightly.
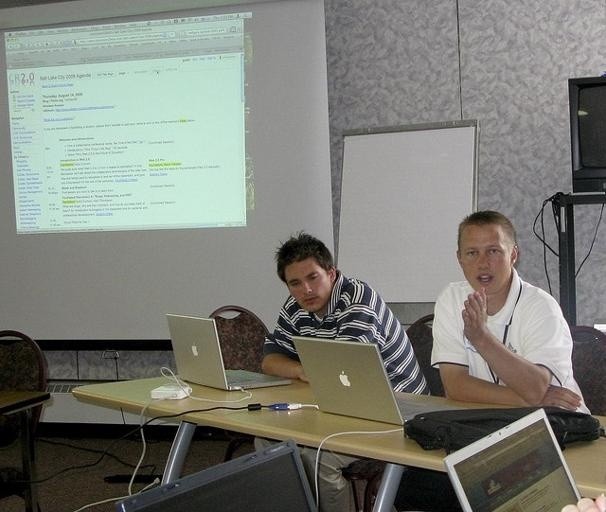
[0,331,42,512]
[400,312,606,415]
[203,306,279,371]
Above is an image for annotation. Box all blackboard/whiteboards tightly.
[338,117,480,318]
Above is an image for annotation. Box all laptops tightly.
[442,407,582,512]
[166,313,292,390]
[291,335,428,425]
[115,439,319,512]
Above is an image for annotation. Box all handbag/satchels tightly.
[400,401,603,459]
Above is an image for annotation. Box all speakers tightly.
[566,77,605,190]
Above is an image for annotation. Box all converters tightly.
[151,385,191,400]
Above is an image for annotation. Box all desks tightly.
[0,387,51,512]
[69,372,602,512]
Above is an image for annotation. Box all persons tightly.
[561,492,606,512]
[252,228,434,512]
[428,208,596,422]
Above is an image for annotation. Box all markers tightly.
[105,474,160,486]
